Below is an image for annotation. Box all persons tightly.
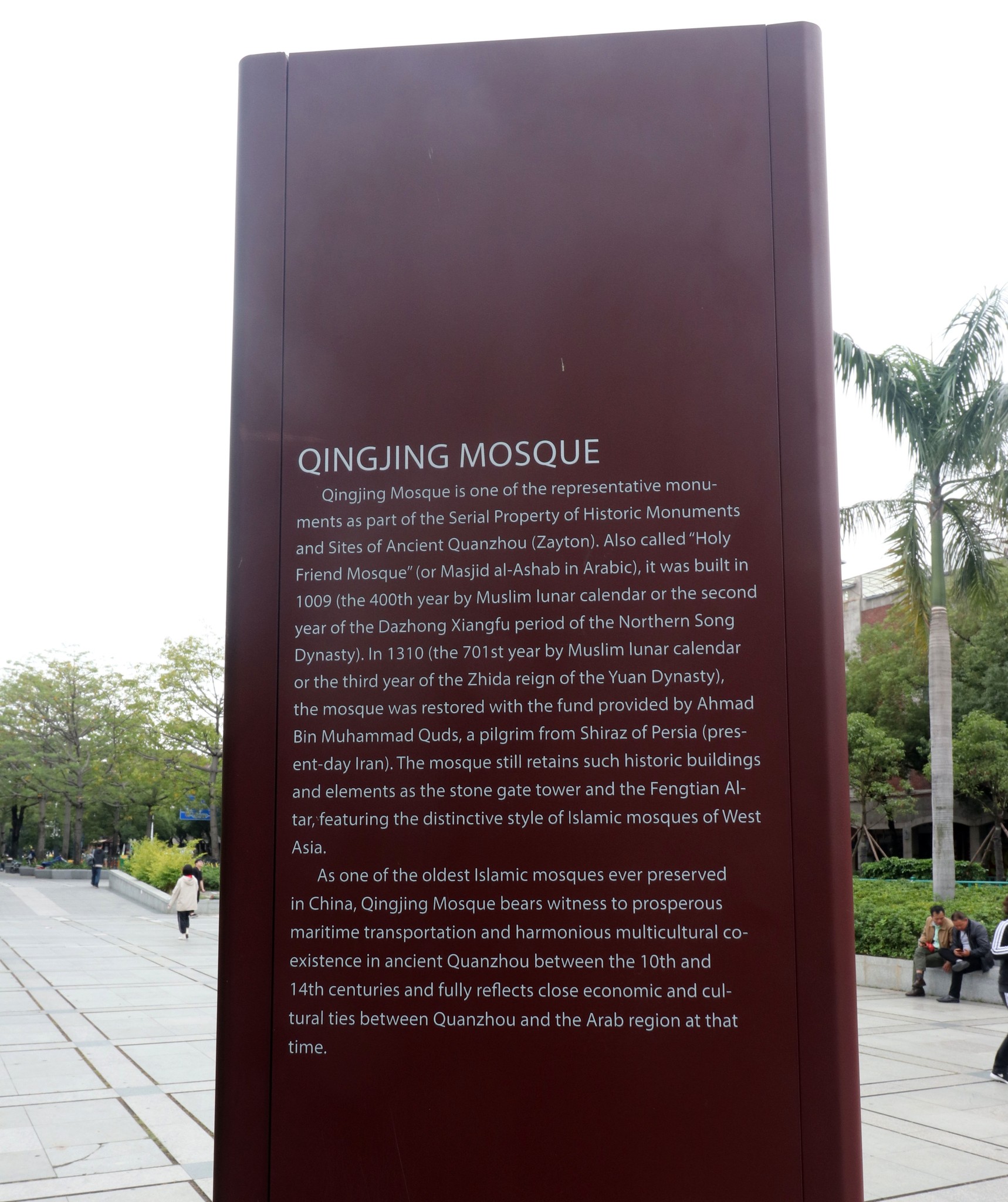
[905,905,955,997]
[189,858,206,917]
[167,864,198,941]
[990,896,1008,1084]
[936,911,995,1003]
[91,842,107,888]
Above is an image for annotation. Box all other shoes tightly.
[952,961,969,972]
[186,933,189,939]
[190,913,199,918]
[912,976,926,987]
[905,988,926,996]
[936,994,959,1003]
[178,937,186,940]
[990,1071,1008,1084]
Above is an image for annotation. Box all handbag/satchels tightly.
[88,857,93,866]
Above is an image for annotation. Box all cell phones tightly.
[956,950,964,952]
